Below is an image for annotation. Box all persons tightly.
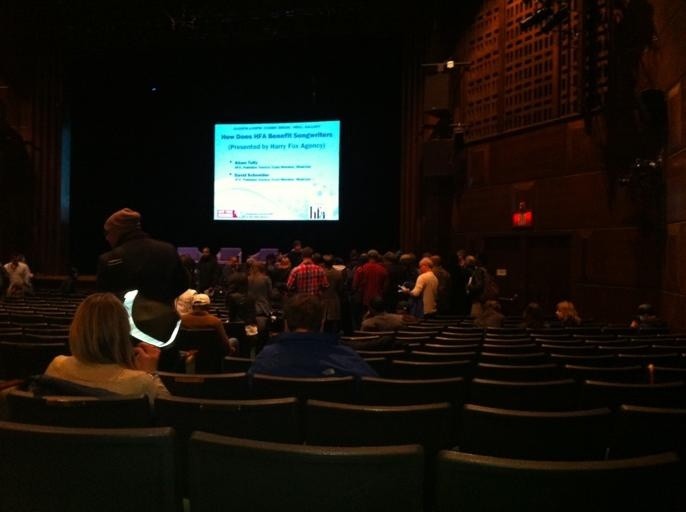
[96,207,186,307]
[178,239,505,331]
[247,294,380,378]
[56,267,78,294]
[631,303,668,330]
[225,272,268,354]
[0,251,32,297]
[520,301,545,328]
[119,261,183,347]
[172,293,240,356]
[555,300,584,326]
[43,291,171,399]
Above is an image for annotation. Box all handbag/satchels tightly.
[115,287,183,350]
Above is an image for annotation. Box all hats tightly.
[103,208,141,232]
[191,294,211,305]
[631,303,657,315]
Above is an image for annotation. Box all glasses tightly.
[101,227,120,237]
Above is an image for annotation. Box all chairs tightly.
[216,248,243,267]
[250,248,279,266]
[177,247,204,267]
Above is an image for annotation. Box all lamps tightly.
[534,8,569,36]
[520,7,552,32]
